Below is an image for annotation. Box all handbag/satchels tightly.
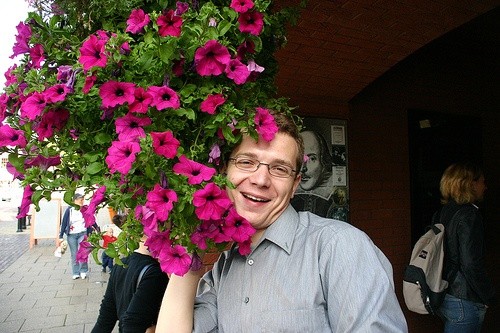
[54,239,67,257]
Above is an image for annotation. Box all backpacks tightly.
[403,201,467,315]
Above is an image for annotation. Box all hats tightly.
[73,193,84,199]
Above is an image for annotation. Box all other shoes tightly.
[102,269,106,272]
[108,270,111,274]
[81,272,87,279]
[73,275,79,279]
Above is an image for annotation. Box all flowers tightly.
[0,0,310,278]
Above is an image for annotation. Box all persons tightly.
[90,234,170,333]
[433,157,500,333]
[101,228,117,274]
[155,110,408,333]
[59,193,101,280]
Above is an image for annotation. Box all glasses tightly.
[228,156,296,178]
